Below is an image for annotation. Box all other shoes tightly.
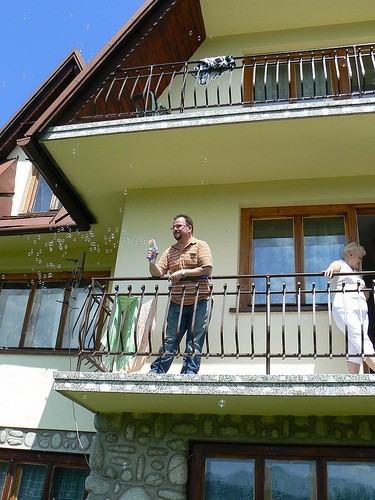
[148,369,161,373]
[182,369,196,374]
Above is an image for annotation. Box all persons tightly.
[147,213,213,374]
[321,242,375,375]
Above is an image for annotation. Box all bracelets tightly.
[181,271,186,279]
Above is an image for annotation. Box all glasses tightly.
[170,224,189,230]
